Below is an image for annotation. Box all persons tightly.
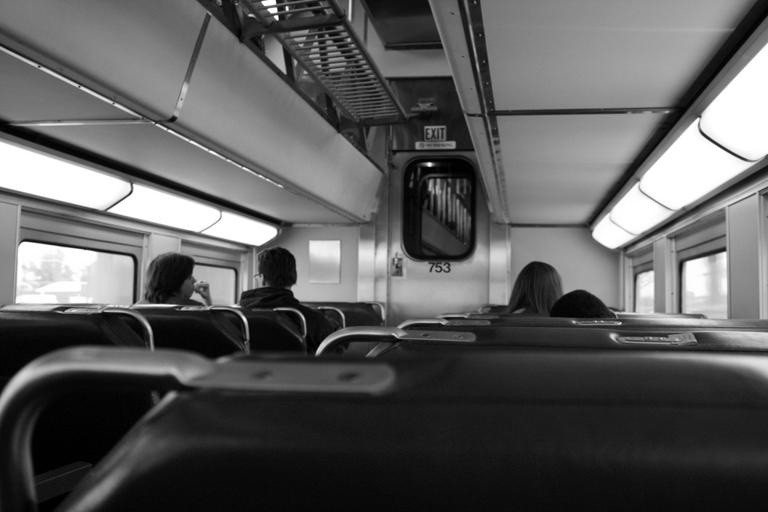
[549,289,616,319]
[498,261,566,318]
[237,246,344,354]
[144,253,214,307]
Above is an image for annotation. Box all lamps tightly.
[0,132,284,251]
[586,12,767,252]
